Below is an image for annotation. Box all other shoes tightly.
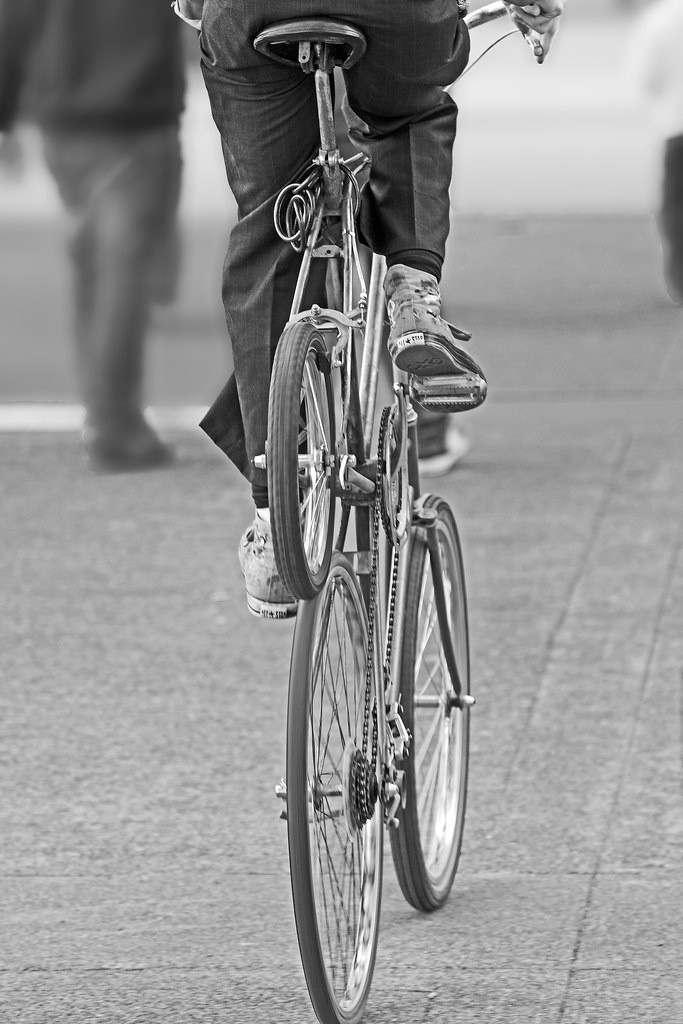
[84,432,175,472]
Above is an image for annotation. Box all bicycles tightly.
[248,0,548,1024]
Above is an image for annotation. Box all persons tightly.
[0,1,185,471]
[163,0,564,617]
[631,1,683,309]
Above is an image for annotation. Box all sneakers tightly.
[416,426,466,477]
[236,510,302,618]
[382,263,485,413]
